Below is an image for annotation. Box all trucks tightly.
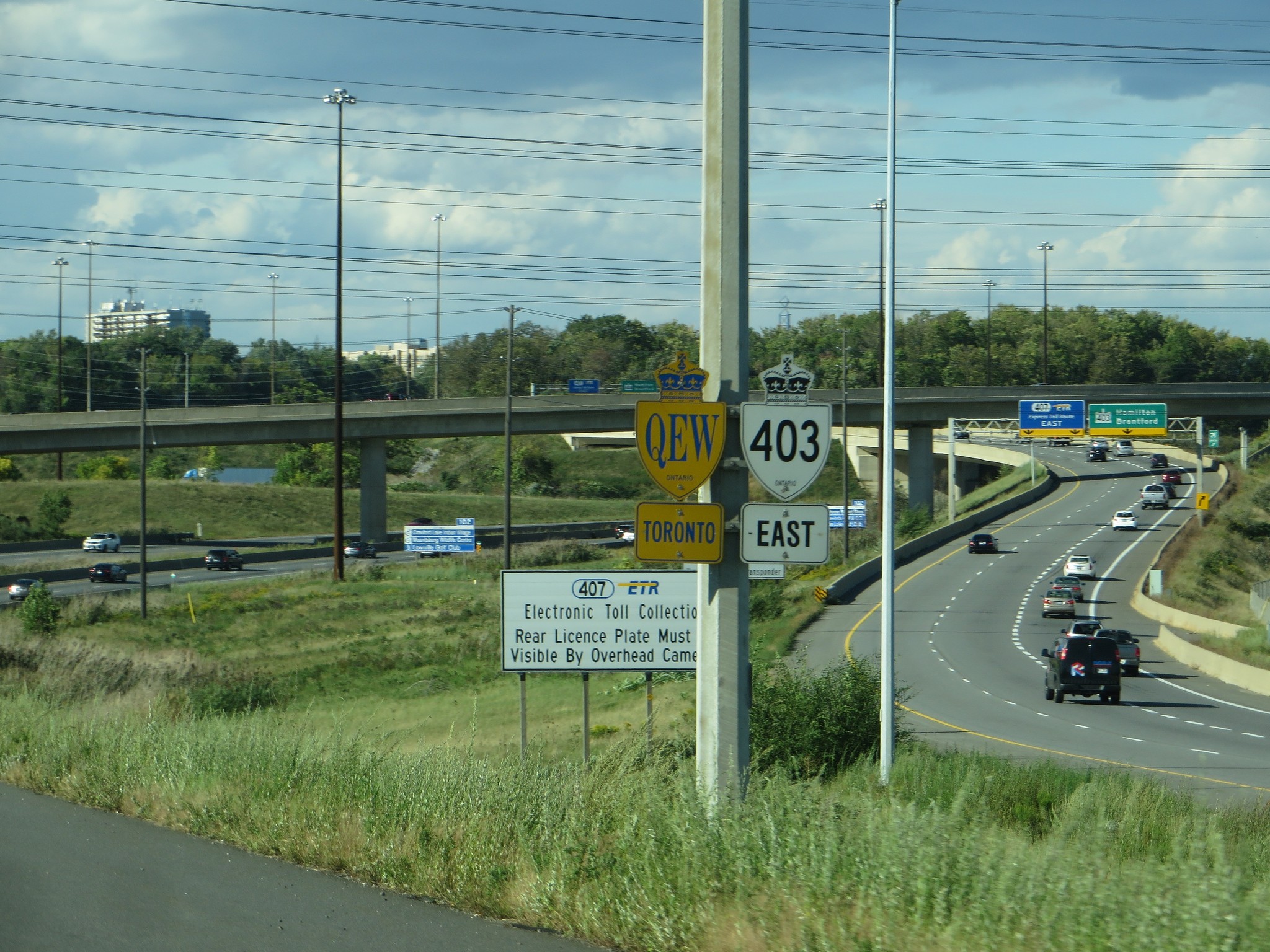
[183,467,275,485]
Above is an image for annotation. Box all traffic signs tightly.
[1018,400,1086,437]
[1088,403,1168,437]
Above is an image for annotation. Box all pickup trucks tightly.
[364,392,417,401]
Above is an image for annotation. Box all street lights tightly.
[268,272,279,404]
[403,296,414,394]
[868,198,886,388]
[82,239,98,412]
[1036,241,1054,383]
[51,256,70,412]
[981,279,996,386]
[430,213,447,399]
[323,87,356,584]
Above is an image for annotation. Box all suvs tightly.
[205,548,244,571]
[82,532,121,553]
[1040,636,1121,705]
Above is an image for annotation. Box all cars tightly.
[343,542,377,559]
[615,524,635,542]
[1015,434,1182,619]
[954,428,970,440]
[88,562,127,583]
[405,518,436,525]
[8,579,44,600]
[967,534,999,554]
[1060,618,1103,637]
[411,551,451,558]
[1092,629,1141,676]
